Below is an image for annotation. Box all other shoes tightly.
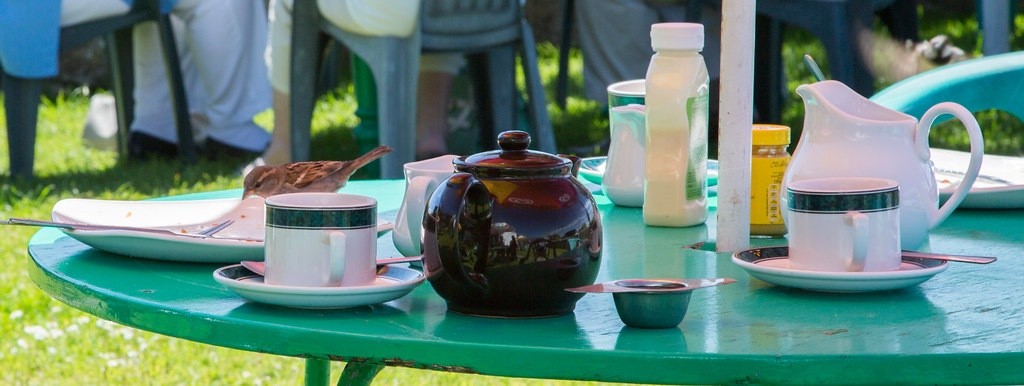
[205,118,276,160]
[127,126,179,160]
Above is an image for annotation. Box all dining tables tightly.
[24,161,1023,386]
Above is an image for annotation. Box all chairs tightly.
[290,0,559,181]
[0,0,199,192]
[858,50,1024,157]
[639,0,1011,153]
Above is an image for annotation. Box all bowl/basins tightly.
[563,278,738,329]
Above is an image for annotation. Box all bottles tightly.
[750,124,791,238]
[642,23,710,228]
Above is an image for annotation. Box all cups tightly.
[602,79,646,207]
[262,193,378,287]
[786,178,903,272]
[392,154,464,267]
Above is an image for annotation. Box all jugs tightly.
[777,80,985,252]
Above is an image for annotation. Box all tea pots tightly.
[419,129,601,319]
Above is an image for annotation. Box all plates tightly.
[927,146,1024,210]
[51,199,396,265]
[212,264,426,309]
[730,246,949,291]
[577,156,719,196]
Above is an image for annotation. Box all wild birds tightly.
[242,144,396,200]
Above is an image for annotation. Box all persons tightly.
[0,0,273,162]
[571,0,725,159]
[244,1,465,175]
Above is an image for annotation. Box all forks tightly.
[4,218,239,240]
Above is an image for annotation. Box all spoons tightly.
[240,254,425,277]
[900,249,997,265]
